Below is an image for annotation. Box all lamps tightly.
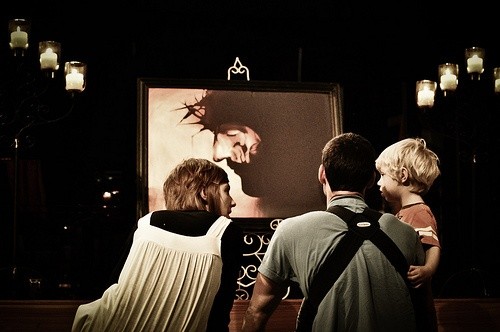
[8,18,88,100]
[415,46,500,112]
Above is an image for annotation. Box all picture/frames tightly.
[136,78,343,229]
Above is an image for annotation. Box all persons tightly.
[72,158,243,332]
[374,138,441,288]
[241,132,438,332]
